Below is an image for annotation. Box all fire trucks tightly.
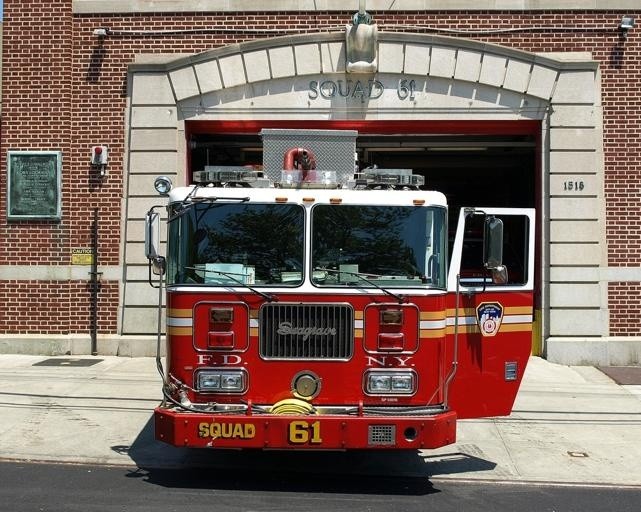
[144,127,538,489]
